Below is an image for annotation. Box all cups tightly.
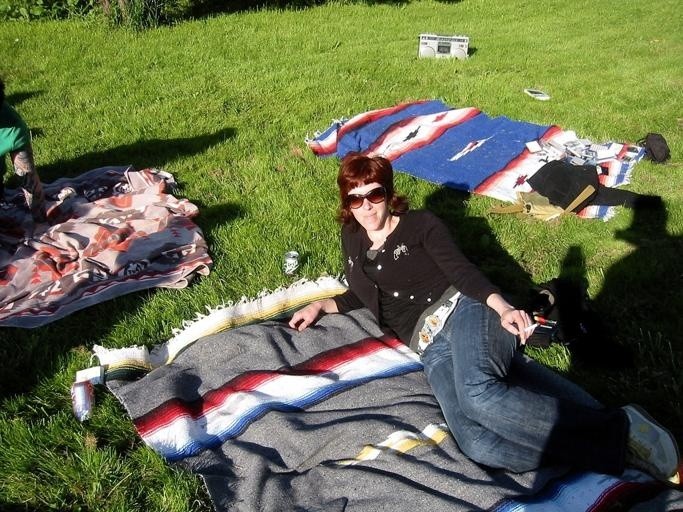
[344,186,387,209]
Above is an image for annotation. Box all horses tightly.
[525,88,551,101]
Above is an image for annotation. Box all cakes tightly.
[72,380,96,422]
[107,228,130,245]
[281,251,302,275]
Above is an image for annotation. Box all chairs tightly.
[619,403,680,478]
[623,438,680,488]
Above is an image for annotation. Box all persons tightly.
[0,79,51,241]
[287,153,683,490]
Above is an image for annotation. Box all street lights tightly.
[635,132,669,164]
